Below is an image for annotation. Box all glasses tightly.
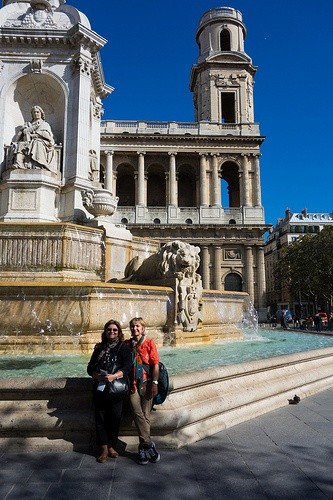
[108,328,117,331]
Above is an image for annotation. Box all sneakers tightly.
[147,441,160,462]
[138,449,148,464]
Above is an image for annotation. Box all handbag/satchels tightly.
[154,361,169,404]
[109,342,129,393]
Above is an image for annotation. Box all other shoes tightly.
[110,453,119,458]
[99,457,107,463]
